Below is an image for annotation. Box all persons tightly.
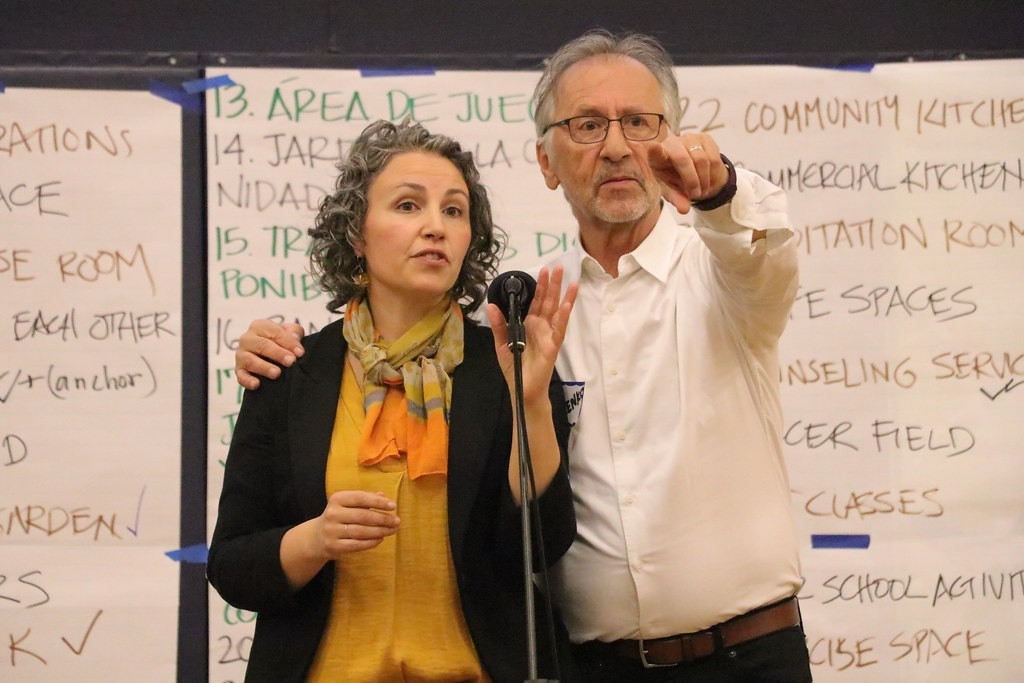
[207,119,577,683]
[236,32,811,683]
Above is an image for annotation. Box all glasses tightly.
[543,113,671,144]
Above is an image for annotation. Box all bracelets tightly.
[691,153,737,211]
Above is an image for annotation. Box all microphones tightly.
[488,270,537,324]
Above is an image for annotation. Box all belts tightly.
[575,596,800,667]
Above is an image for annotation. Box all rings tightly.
[344,524,350,539]
[689,145,703,153]
